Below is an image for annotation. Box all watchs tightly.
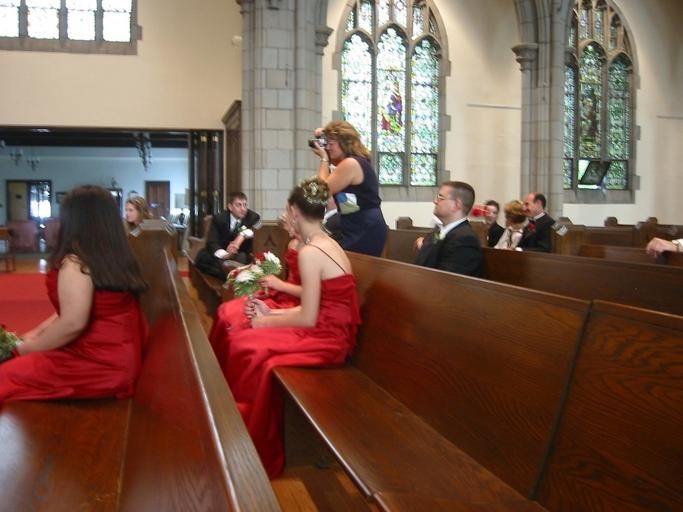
[672,239,681,253]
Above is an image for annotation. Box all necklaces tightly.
[303,230,320,241]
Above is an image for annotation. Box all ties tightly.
[233,222,241,232]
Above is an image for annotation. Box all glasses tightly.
[436,193,455,202]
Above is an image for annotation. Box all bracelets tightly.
[320,159,328,163]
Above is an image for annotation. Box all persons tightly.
[414,181,489,280]
[645,236,683,260]
[125,196,176,324]
[220,176,364,479]
[207,213,307,358]
[1,184,152,401]
[517,192,556,254]
[494,199,525,251]
[483,201,506,247]
[313,120,389,257]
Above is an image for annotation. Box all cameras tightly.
[308,134,327,149]
